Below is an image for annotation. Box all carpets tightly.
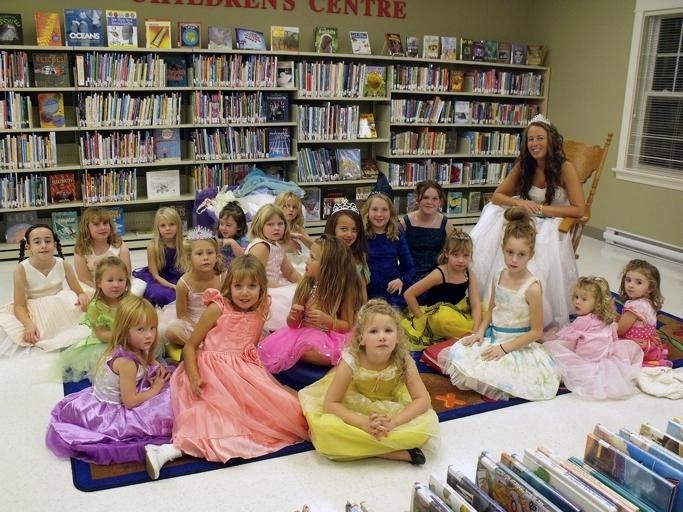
[58,289,682,497]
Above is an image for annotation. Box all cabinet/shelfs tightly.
[292,49,393,244]
[0,41,198,261]
[189,46,298,204]
[385,55,551,231]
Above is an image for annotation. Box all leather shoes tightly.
[407,447,426,465]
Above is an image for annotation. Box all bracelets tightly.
[498,343,508,356]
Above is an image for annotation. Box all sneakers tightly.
[143,443,163,480]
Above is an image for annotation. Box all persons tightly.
[437,203,562,400]
[144,255,303,480]
[296,297,441,465]
[47,295,179,466]
[466,110,590,337]
[544,274,643,401]
[613,257,682,397]
[0,170,482,374]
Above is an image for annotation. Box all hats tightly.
[367,171,395,204]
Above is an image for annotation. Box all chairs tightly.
[559,131,614,259]
[191,185,280,231]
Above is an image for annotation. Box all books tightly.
[0,7,546,248]
[302,416,682,511]
